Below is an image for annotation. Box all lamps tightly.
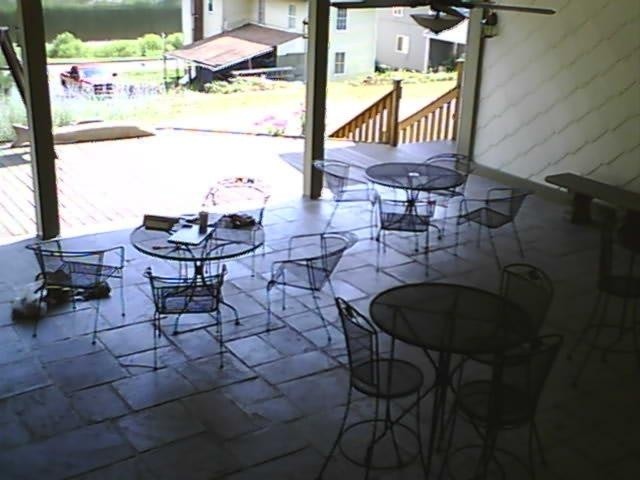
[479,9,499,38]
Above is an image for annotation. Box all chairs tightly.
[201,174,271,277]
[569,218,640,384]
[455,186,538,270]
[267,229,359,342]
[26,239,126,346]
[365,152,478,277]
[316,160,381,241]
[316,263,563,478]
[144,264,229,372]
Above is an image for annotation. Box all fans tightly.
[330,0,556,36]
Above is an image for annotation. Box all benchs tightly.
[542,172,640,236]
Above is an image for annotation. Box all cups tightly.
[198,211,208,235]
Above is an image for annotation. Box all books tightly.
[167,223,216,247]
[144,213,181,230]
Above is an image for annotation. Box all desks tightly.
[130,211,266,329]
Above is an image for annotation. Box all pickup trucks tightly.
[60,61,118,103]
